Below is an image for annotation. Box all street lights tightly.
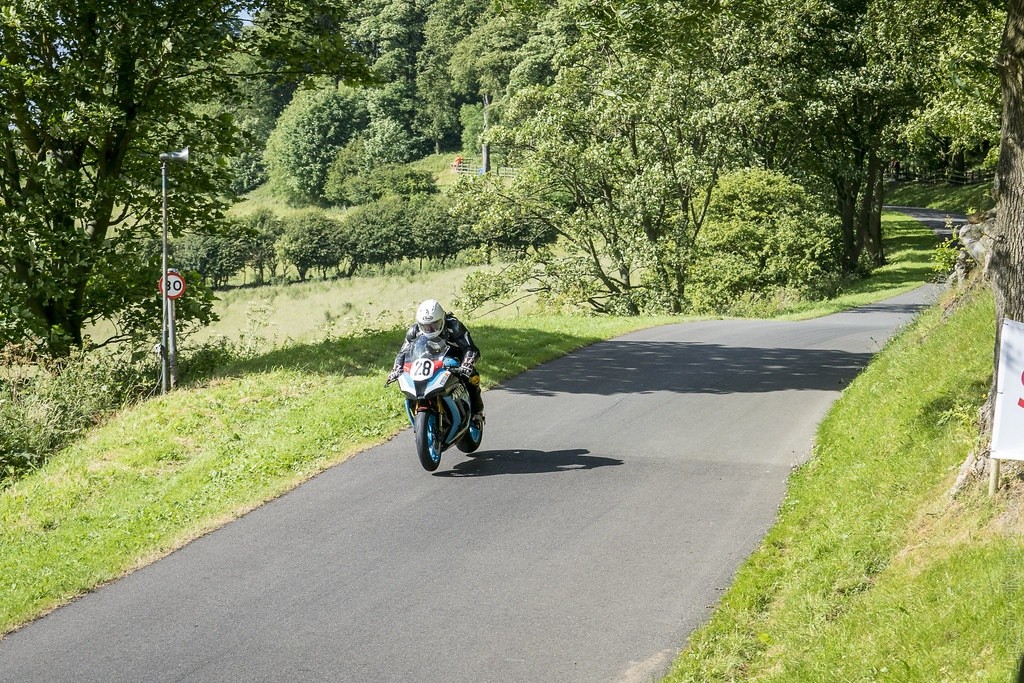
[159,146,189,394]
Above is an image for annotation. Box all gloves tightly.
[460,361,474,376]
[388,368,403,381]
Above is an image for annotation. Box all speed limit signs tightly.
[159,272,185,299]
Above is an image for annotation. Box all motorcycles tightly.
[384,334,487,471]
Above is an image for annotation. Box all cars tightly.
[453,158,477,170]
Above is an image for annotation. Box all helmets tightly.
[416,299,445,339]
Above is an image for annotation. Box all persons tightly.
[455,155,461,164]
[388,299,484,431]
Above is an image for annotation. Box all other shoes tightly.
[473,410,484,428]
